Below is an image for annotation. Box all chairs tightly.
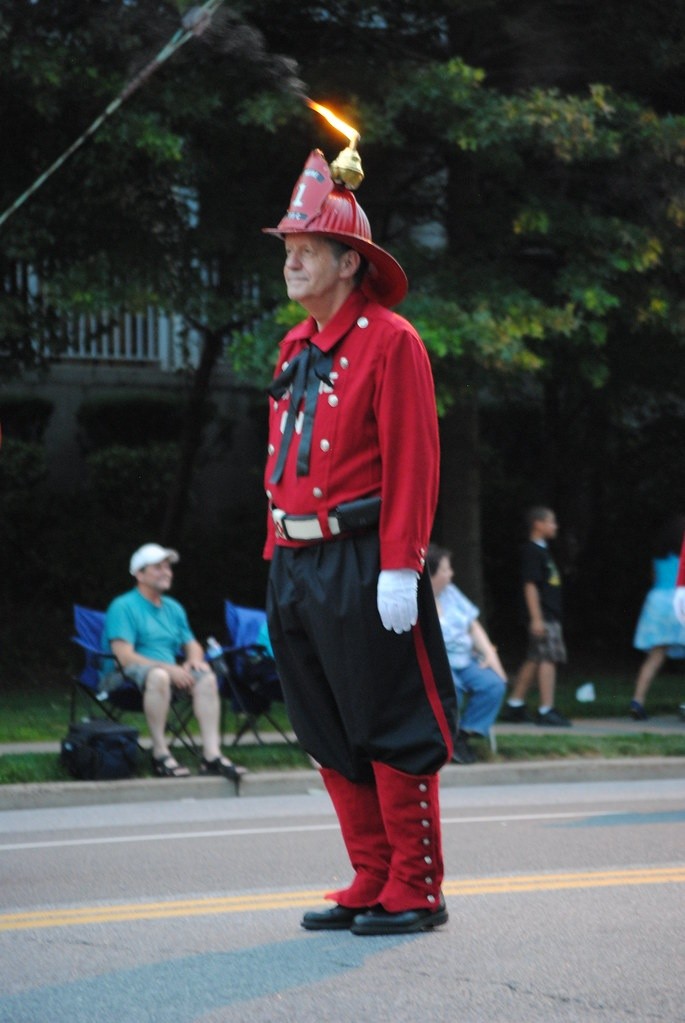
[63,604,202,760]
[205,599,298,765]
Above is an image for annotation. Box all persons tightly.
[501,508,574,726]
[673,537,685,626]
[424,541,509,763]
[251,143,460,936]
[97,543,247,778]
[625,540,685,722]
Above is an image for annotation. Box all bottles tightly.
[206,637,228,676]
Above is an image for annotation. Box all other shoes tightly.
[628,700,648,721]
[532,707,572,728]
[501,702,533,722]
[452,745,473,765]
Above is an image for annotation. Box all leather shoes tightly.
[300,904,376,930]
[349,890,449,934]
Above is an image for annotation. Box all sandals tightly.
[195,754,248,777]
[150,754,192,777]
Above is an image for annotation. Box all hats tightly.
[128,542,181,576]
[261,135,409,308]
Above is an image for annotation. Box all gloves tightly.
[376,567,421,635]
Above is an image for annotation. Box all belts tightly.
[267,504,340,541]
